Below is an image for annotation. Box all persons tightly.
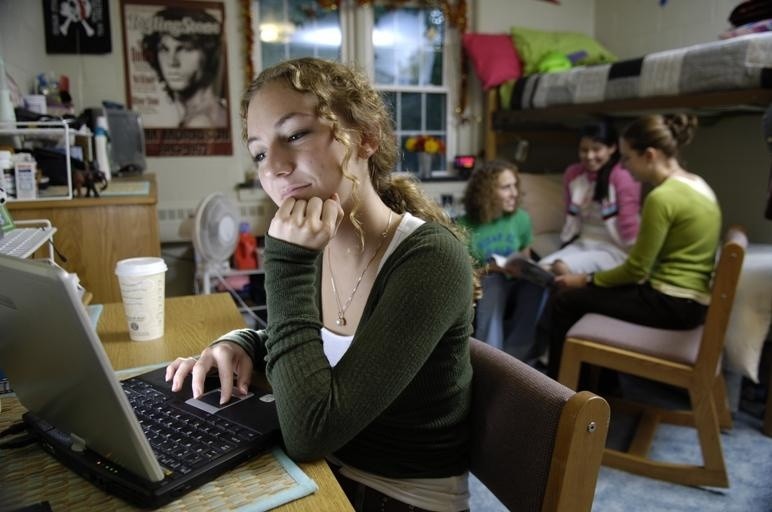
[446,159,548,363]
[166,57,477,511]
[141,10,228,128]
[539,119,642,298]
[549,110,722,379]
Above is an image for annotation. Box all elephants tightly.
[72,169,109,198]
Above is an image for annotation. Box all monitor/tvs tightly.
[69,108,146,178]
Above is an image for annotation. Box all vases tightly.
[417,151,433,180]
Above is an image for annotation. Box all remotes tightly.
[102,102,124,110]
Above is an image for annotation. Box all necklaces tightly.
[326,211,394,325]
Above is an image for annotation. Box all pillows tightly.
[462,32,523,91]
[509,23,621,77]
[515,172,571,235]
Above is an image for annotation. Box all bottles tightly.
[24,93,47,115]
[1,89,17,132]
[15,151,37,201]
[0,151,17,199]
[95,125,113,182]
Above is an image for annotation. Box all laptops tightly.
[0,254,284,511]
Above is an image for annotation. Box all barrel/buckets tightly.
[232,221,257,271]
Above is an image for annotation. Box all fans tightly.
[192,190,240,275]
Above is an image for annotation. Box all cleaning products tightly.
[95,115,114,184]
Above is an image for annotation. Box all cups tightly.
[113,256,169,342]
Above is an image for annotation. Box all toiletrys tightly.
[36,72,49,95]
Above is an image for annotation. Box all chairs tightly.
[465,331,610,512]
[559,223,749,489]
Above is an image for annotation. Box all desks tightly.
[0,291,355,512]
[191,268,268,327]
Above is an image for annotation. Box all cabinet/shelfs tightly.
[0,173,162,306]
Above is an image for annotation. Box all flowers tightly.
[405,134,446,157]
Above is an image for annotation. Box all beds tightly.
[482,24,772,436]
